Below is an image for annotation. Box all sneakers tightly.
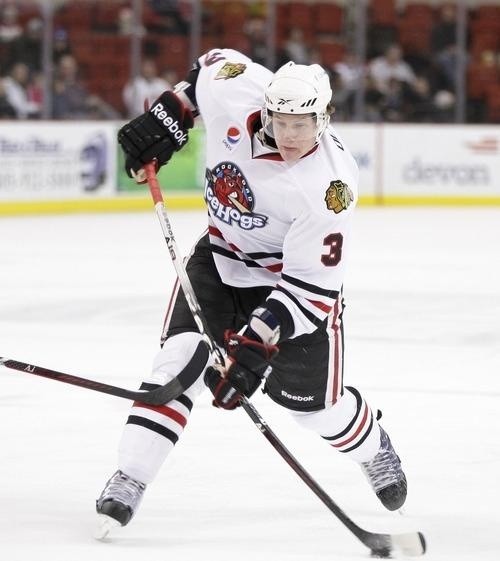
[96,470,146,525]
[360,424,407,511]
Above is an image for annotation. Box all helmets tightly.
[261,60,332,141]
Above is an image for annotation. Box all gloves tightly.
[203,329,279,410]
[118,91,194,178]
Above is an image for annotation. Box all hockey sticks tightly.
[0,340,210,407]
[135,96,427,560]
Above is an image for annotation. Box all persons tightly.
[97,46,406,527]
